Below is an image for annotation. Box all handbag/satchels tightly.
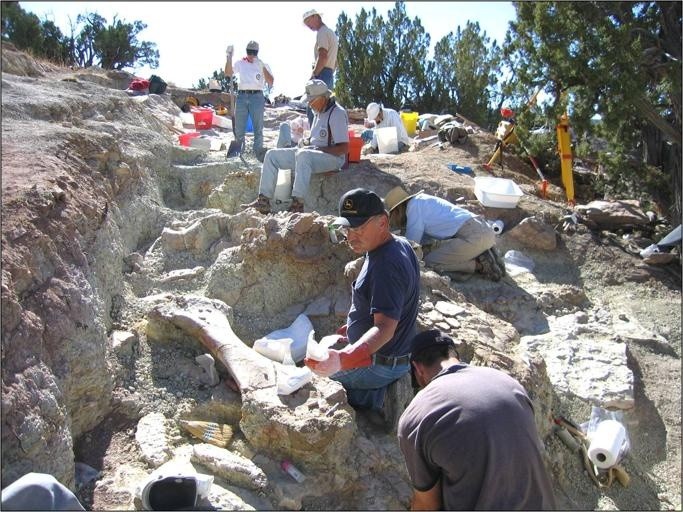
[125,75,167,96]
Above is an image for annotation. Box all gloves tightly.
[226,45,233,56]
[304,342,372,378]
[337,325,348,342]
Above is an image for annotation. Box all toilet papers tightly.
[588,420,625,469]
[492,220,504,234]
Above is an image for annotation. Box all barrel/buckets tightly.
[374,127,399,154]
[400,112,419,135]
[191,107,214,130]
[177,131,202,146]
[348,137,363,162]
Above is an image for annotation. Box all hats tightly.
[303,8,324,21]
[331,186,425,228]
[366,103,380,121]
[410,330,455,387]
[300,79,328,105]
[247,40,258,56]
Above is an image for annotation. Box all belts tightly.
[374,355,409,367]
[239,90,261,94]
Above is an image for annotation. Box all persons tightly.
[385,187,507,284]
[224,42,275,154]
[360,102,410,157]
[239,80,349,212]
[302,186,420,432]
[399,330,557,511]
[303,9,338,131]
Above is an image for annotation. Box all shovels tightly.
[226,46,245,158]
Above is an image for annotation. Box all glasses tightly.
[337,217,374,237]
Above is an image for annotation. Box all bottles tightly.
[281,460,306,484]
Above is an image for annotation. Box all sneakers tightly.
[239,194,271,215]
[288,198,304,212]
[477,249,506,282]
[382,373,414,433]
